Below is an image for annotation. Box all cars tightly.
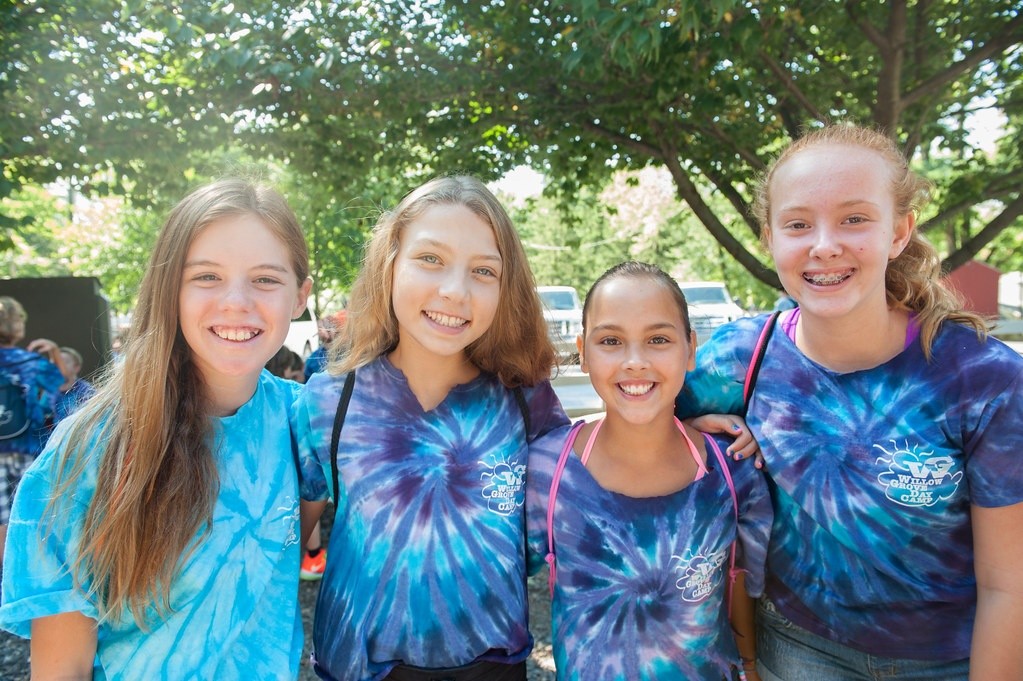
[284,306,322,363]
[534,286,586,365]
[678,282,743,336]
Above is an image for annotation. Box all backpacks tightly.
[0,364,32,439]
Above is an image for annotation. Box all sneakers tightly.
[299,548,327,582]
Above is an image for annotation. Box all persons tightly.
[294,171,767,681]
[0,295,67,582]
[303,315,342,384]
[0,179,315,681]
[40,344,91,442]
[523,261,777,681]
[676,124,1023,681]
[263,348,331,581]
